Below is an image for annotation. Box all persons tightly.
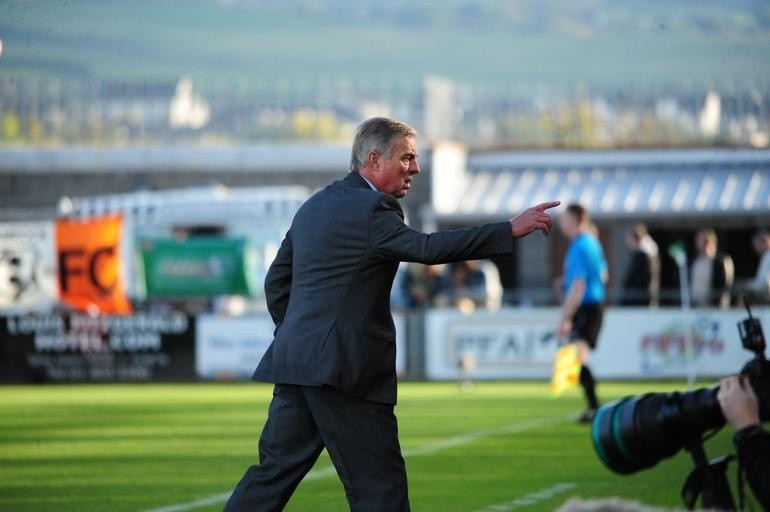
[687,228,734,308]
[735,228,769,305]
[716,371,769,510]
[618,222,661,309]
[438,257,505,311]
[559,203,610,422]
[391,262,453,311]
[222,116,561,512]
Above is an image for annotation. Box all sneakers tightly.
[576,407,596,424]
